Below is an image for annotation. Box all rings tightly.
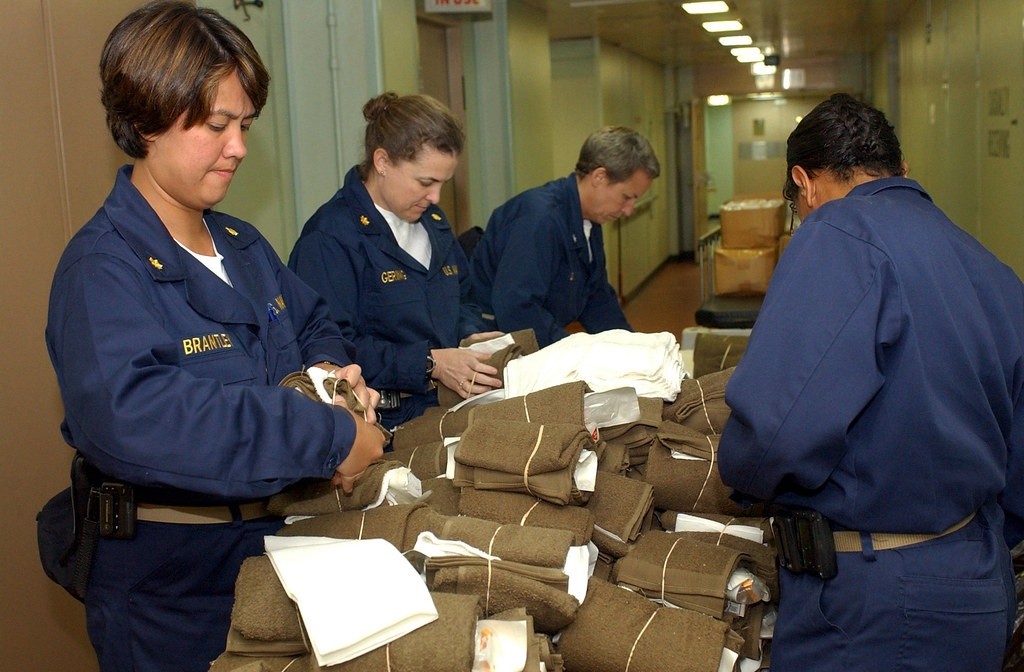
[459,379,468,389]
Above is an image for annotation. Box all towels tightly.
[208,327,781,672]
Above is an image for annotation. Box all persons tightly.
[472,126,660,348]
[717,92,1024,672]
[46,0,384,671]
[289,92,484,454]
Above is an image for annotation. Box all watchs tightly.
[426,348,436,380]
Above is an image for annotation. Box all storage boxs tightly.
[713,200,795,294]
